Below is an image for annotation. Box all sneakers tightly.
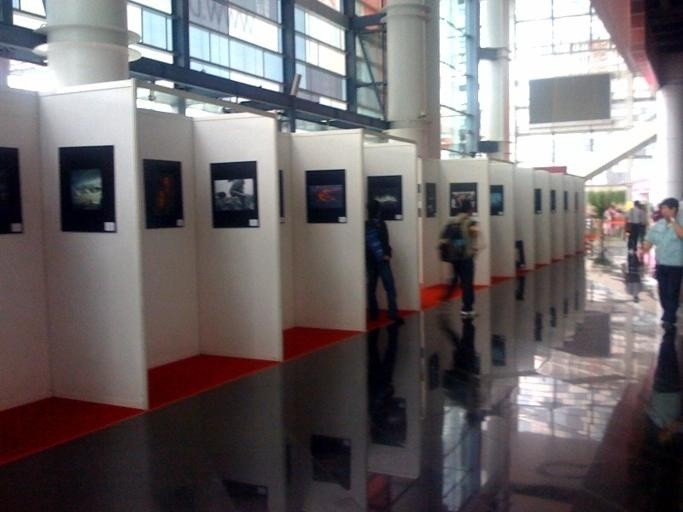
[459,309,476,317]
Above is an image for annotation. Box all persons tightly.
[621,251,643,303]
[435,312,486,428]
[367,322,410,470]
[652,202,663,221]
[623,200,644,253]
[441,199,480,317]
[643,324,682,442]
[366,197,404,324]
[638,203,648,250]
[635,197,682,324]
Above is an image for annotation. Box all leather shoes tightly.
[386,310,404,326]
[662,313,676,335]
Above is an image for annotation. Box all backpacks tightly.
[439,224,464,262]
[364,225,382,258]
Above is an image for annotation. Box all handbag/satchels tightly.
[624,222,632,233]
